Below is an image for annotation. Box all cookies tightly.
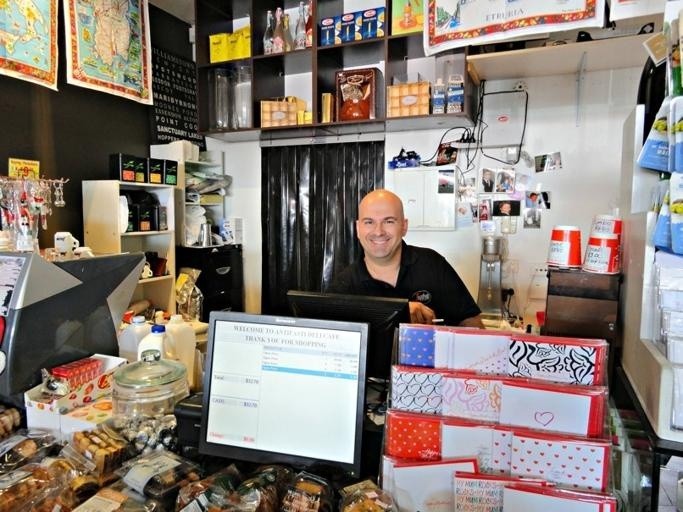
[75,424,128,473]
[0,407,20,438]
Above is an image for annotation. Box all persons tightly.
[498,202,512,215]
[527,192,537,208]
[328,189,485,329]
[483,169,494,191]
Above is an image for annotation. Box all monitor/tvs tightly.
[198,310,369,478]
[287,288,412,391]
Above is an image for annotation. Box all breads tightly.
[0,427,54,478]
[0,460,98,512]
[295,477,325,498]
[344,499,384,511]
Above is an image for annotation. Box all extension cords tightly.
[449,141,480,149]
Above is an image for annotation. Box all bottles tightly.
[263,9,272,56]
[305,1,312,48]
[294,1,306,51]
[113,349,189,428]
[281,14,292,52]
[271,7,285,55]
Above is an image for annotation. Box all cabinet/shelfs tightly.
[395,166,456,231]
[196,243,246,322]
[620,106,683,512]
[151,142,225,249]
[118,182,176,325]
[543,270,622,383]
[194,0,467,135]
